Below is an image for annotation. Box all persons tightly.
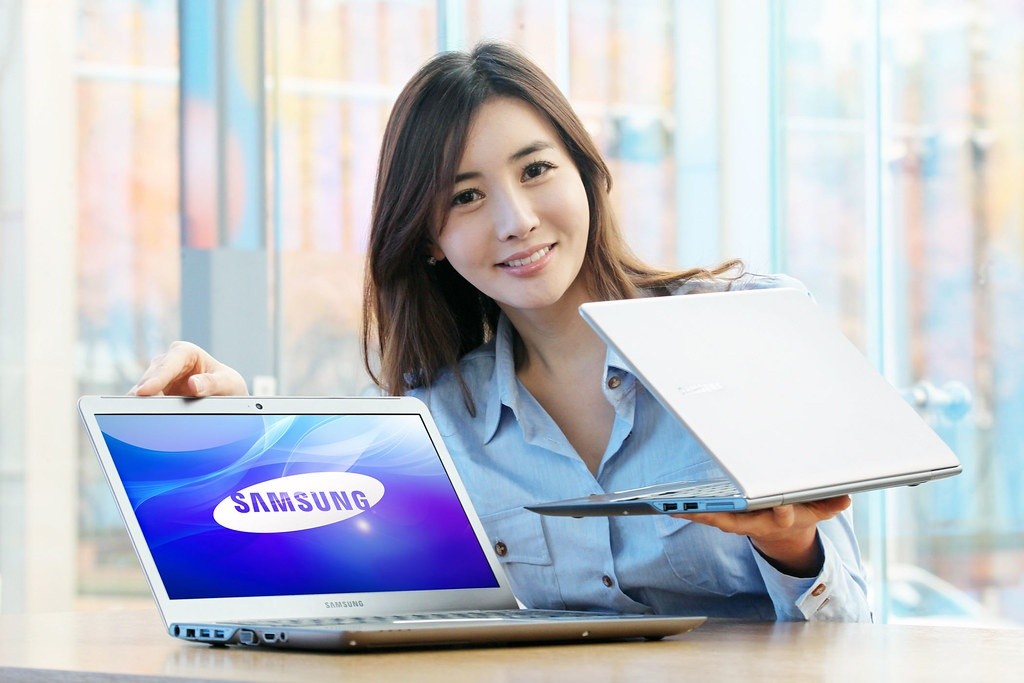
[127,44,875,622]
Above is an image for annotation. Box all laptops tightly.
[77,393,709,654]
[524,287,962,518]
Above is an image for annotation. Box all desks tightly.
[0,598,1023,683]
